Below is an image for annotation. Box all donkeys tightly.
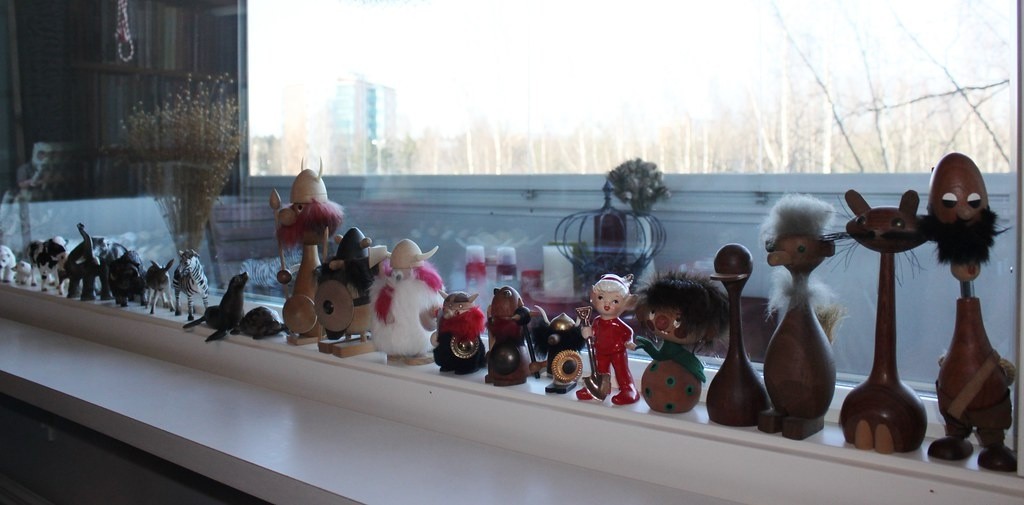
[145,258,175,316]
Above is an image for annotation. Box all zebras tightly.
[172,249,210,322]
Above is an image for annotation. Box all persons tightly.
[628,268,730,416]
[575,271,642,406]
[926,151,1020,473]
[16,138,63,200]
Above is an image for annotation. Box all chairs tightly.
[207,205,299,297]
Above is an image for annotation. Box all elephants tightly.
[64,222,117,302]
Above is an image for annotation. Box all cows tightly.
[27,236,69,293]
